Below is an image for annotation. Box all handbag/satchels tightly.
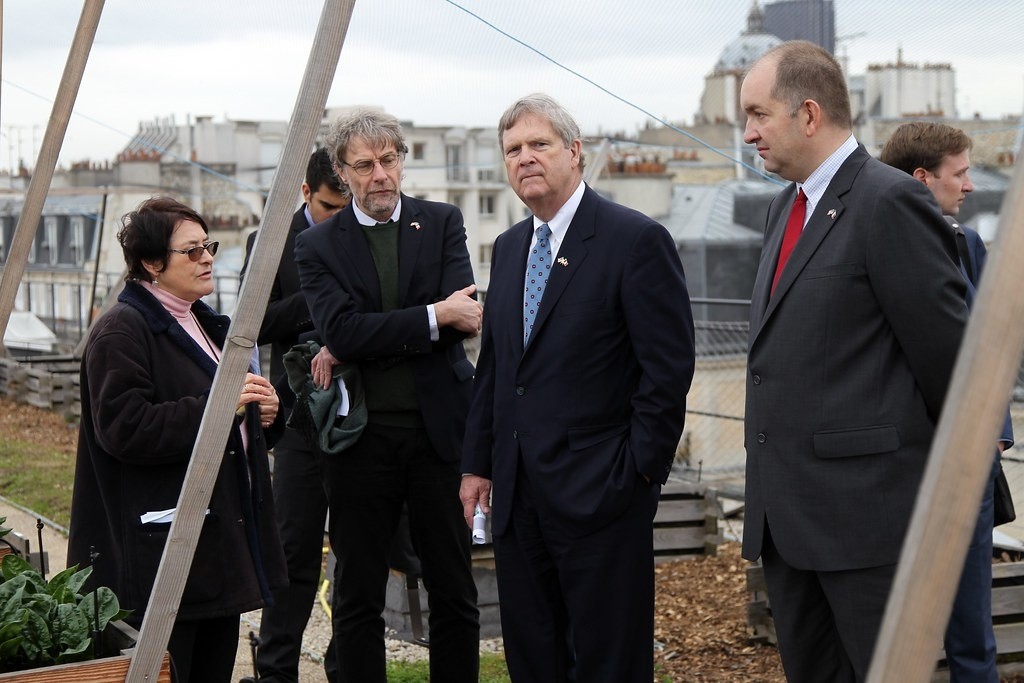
[992,454,1016,527]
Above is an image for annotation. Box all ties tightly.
[524,225,552,347]
[769,187,807,301]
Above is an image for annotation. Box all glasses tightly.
[168,241,219,262]
[341,149,400,176]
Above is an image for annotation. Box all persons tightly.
[239,146,352,683]
[878,122,1018,683]
[741,39,973,683]
[297,106,482,683]
[64,192,287,683]
[457,91,695,683]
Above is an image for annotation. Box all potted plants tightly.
[0,477,171,683]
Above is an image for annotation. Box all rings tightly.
[267,422,270,428]
